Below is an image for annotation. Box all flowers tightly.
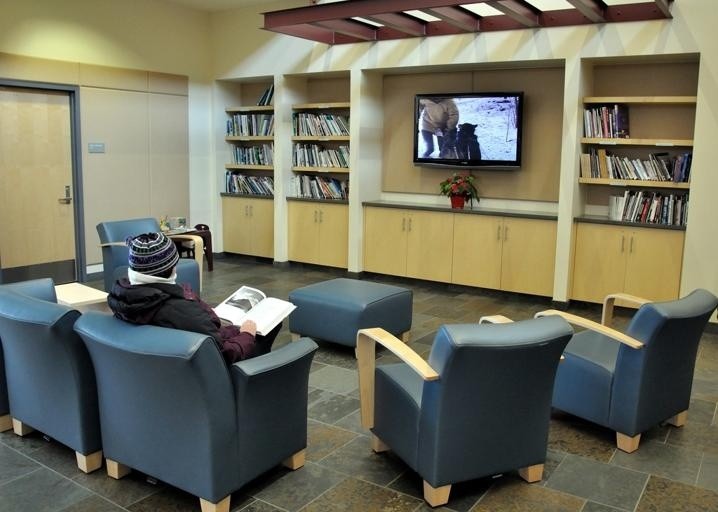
[439,173,480,210]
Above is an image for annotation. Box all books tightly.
[225,170,274,195]
[580,147,692,182]
[608,190,689,226]
[232,142,274,166]
[583,105,630,139]
[226,113,274,136]
[292,111,350,136]
[292,173,349,200]
[212,285,298,336]
[292,142,350,168]
[256,84,274,106]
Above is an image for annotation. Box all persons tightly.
[418,98,459,158]
[107,233,282,369]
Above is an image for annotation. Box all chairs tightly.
[0,277,103,474]
[95,217,204,300]
[71,309,319,512]
[534,288,718,454]
[187,224,209,259]
[357,314,575,508]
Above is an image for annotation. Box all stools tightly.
[288,276,413,359]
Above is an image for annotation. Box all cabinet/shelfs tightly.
[577,95,697,189]
[362,201,454,285]
[285,196,349,270]
[223,105,274,170]
[567,214,686,309]
[451,208,558,297]
[220,194,274,259]
[290,103,350,173]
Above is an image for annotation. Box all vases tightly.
[450,193,465,209]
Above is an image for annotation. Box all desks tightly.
[170,229,213,271]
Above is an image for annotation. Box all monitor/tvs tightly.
[412,91,524,171]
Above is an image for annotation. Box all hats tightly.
[125,232,180,276]
